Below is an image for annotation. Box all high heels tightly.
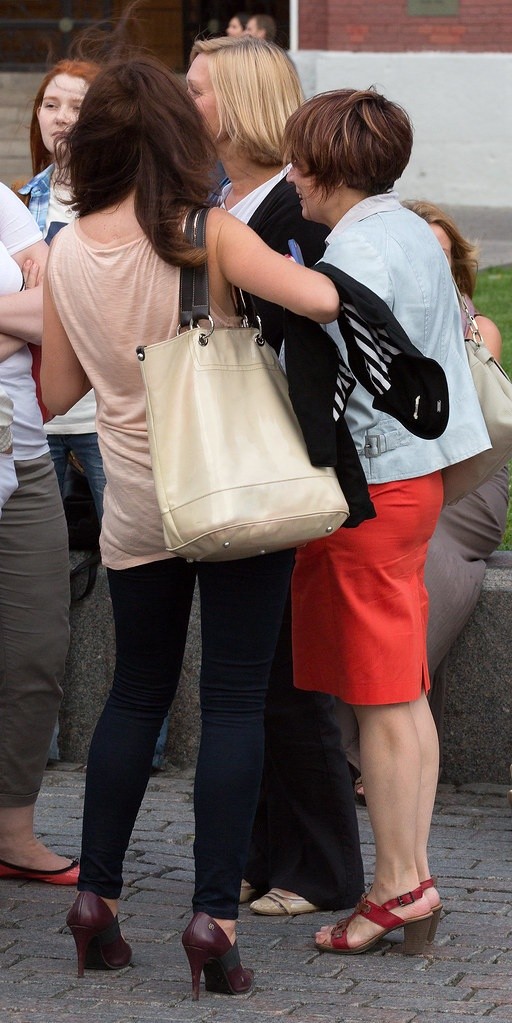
[65,890,133,978]
[311,872,446,955]
[180,911,256,1001]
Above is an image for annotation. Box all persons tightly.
[187,33,367,916]
[337,199,509,805]
[245,15,277,42]
[40,56,341,1004]
[20,60,172,777]
[280,88,493,957]
[0,184,81,885]
[226,14,249,36]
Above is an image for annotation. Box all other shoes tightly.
[352,765,366,806]
[249,886,322,916]
[0,852,80,885]
[237,878,256,904]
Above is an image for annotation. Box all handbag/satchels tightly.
[442,276,512,505]
[135,203,350,563]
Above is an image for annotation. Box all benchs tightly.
[57,550,512,783]
